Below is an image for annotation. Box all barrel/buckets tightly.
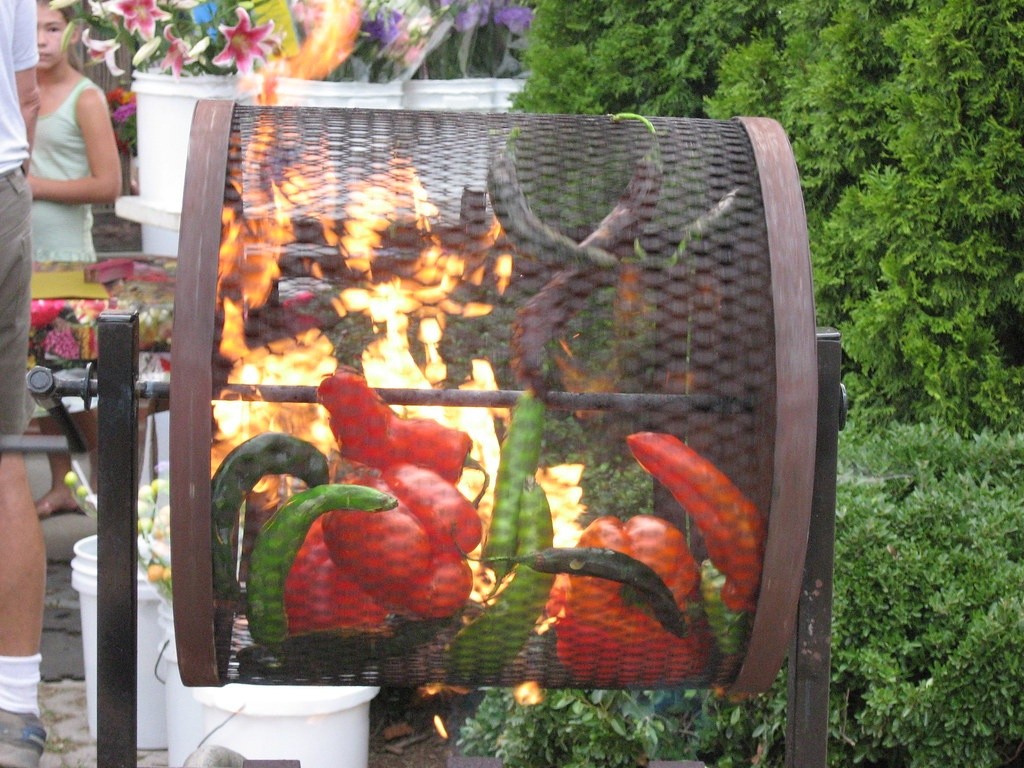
[235,74,402,111]
[69,534,178,750]
[129,70,261,211]
[139,223,179,254]
[153,598,380,768]
[409,76,528,113]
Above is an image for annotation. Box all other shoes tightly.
[34,492,84,519]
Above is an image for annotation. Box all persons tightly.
[0,0,48,768]
[26,0,123,518]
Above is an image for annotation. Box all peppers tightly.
[209,112,769,688]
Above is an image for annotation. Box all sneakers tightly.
[0,709,47,768]
